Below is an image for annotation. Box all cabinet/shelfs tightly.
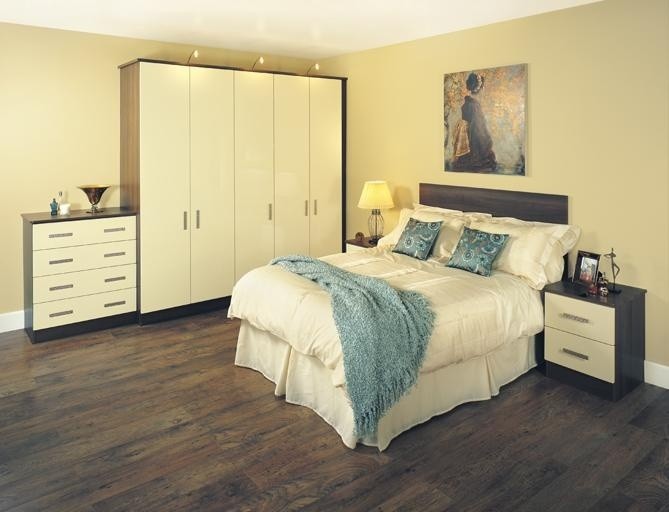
[139,63,234,318]
[273,72,342,271]
[19,205,137,346]
[233,70,275,287]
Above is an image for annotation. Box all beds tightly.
[225,182,569,452]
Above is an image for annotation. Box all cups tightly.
[59,203,72,216]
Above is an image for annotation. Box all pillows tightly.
[444,226,509,279]
[392,217,443,261]
[412,202,492,233]
[543,225,581,285]
[376,208,469,264]
[448,220,570,290]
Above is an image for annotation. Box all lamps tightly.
[356,180,394,243]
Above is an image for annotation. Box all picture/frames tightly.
[572,249,601,295]
[444,63,529,176]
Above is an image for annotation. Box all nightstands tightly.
[344,235,381,253]
[541,277,647,403]
